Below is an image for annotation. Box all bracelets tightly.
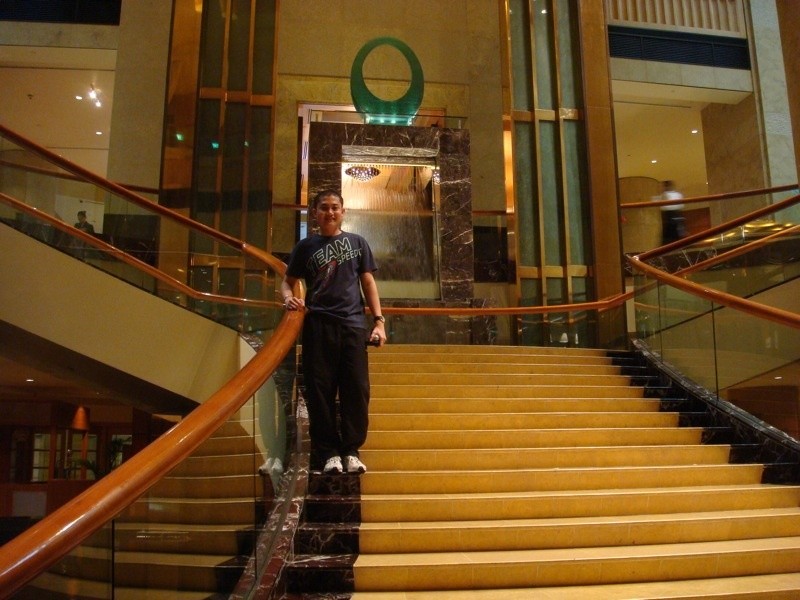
[283,295,291,303]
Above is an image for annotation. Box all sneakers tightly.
[324,456,343,473]
[343,456,367,473]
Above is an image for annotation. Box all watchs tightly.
[374,315,386,323]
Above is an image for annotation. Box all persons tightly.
[658,180,684,241]
[74,211,95,233]
[71,460,96,479]
[281,190,388,473]
